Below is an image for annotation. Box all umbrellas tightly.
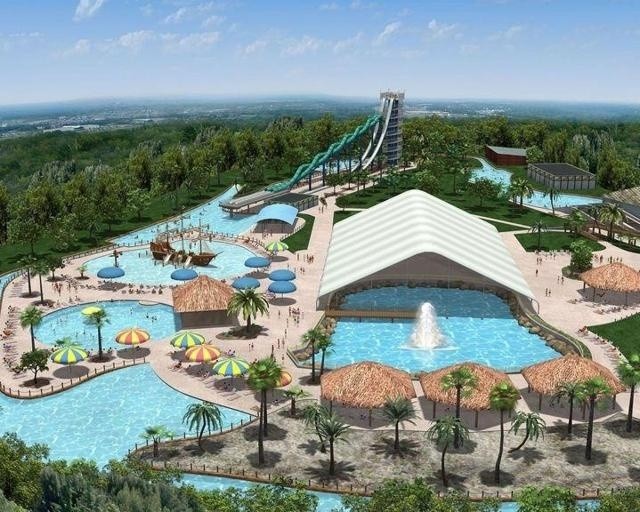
[266,370,293,394]
[170,265,198,283]
[50,345,89,374]
[265,240,289,255]
[184,342,222,367]
[268,268,297,282]
[170,329,205,349]
[268,280,297,300]
[212,355,251,384]
[232,275,261,292]
[115,326,151,352]
[97,266,126,282]
[243,256,271,271]
